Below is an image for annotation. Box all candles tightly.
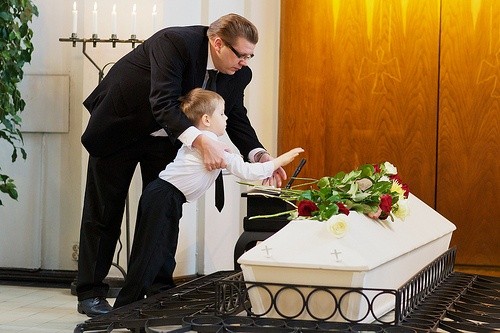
[72,1,78,33]
[92,1,98,34]
[112,3,117,34]
[152,4,157,34]
[131,4,136,34]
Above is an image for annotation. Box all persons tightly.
[113,88,304,310]
[76,14,287,317]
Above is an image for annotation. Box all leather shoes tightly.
[77,297,112,317]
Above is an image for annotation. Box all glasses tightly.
[221,39,254,61]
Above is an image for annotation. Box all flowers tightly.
[235,161,410,224]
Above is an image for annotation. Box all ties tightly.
[209,68,225,212]
